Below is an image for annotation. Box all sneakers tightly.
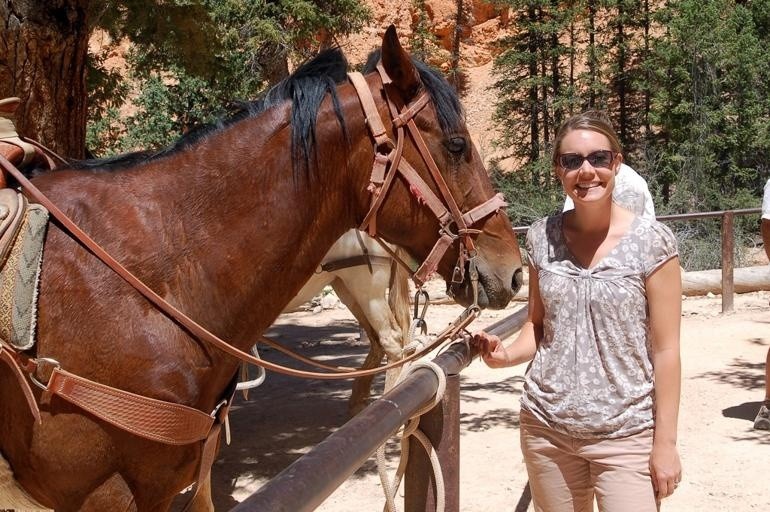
[753,399,770,429]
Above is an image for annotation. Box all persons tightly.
[461,109,688,512]
[753,177,770,432]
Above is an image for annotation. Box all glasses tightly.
[557,149,617,171]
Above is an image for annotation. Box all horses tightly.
[3,26,525,512]
[282,229,419,417]
